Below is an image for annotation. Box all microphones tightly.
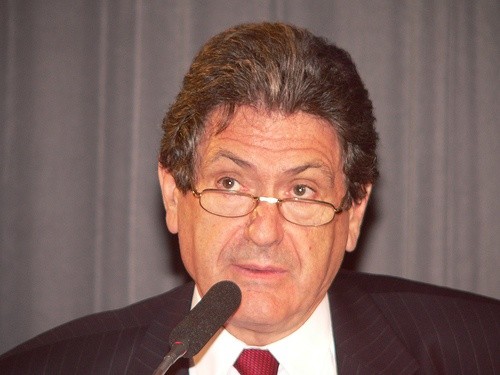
[149,279,244,375]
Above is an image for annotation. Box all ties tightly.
[233,348,279,375]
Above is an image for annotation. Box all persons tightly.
[1,22,499,375]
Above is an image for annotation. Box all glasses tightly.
[186,175,349,228]
